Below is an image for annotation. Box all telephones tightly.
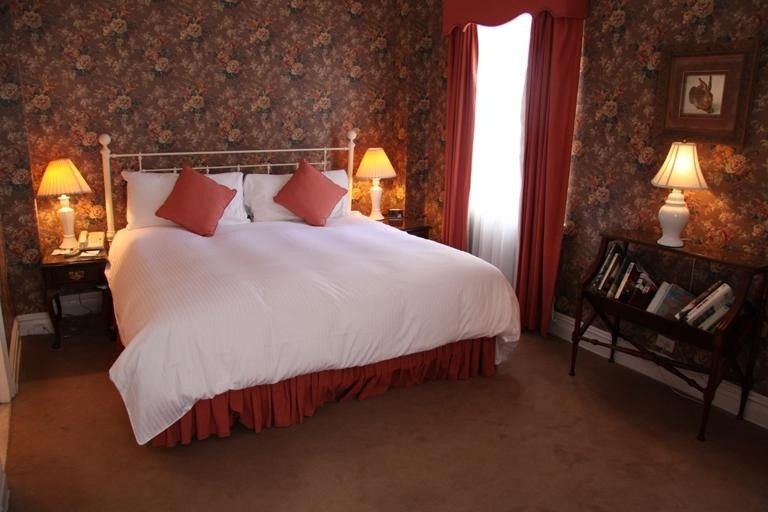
[78,230,105,250]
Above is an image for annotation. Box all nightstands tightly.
[40,249,118,349]
[377,218,431,240]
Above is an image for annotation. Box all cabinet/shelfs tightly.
[568,230,768,442]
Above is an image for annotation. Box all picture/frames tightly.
[653,45,761,145]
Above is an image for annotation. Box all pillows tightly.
[273,159,348,226]
[244,169,349,223]
[120,170,251,230]
[155,166,237,236]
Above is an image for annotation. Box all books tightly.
[593,241,735,367]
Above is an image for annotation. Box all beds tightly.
[99,131,520,450]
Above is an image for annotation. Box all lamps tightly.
[37,159,93,250]
[355,148,397,221]
[651,142,709,248]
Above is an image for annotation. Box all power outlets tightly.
[20,319,53,338]
